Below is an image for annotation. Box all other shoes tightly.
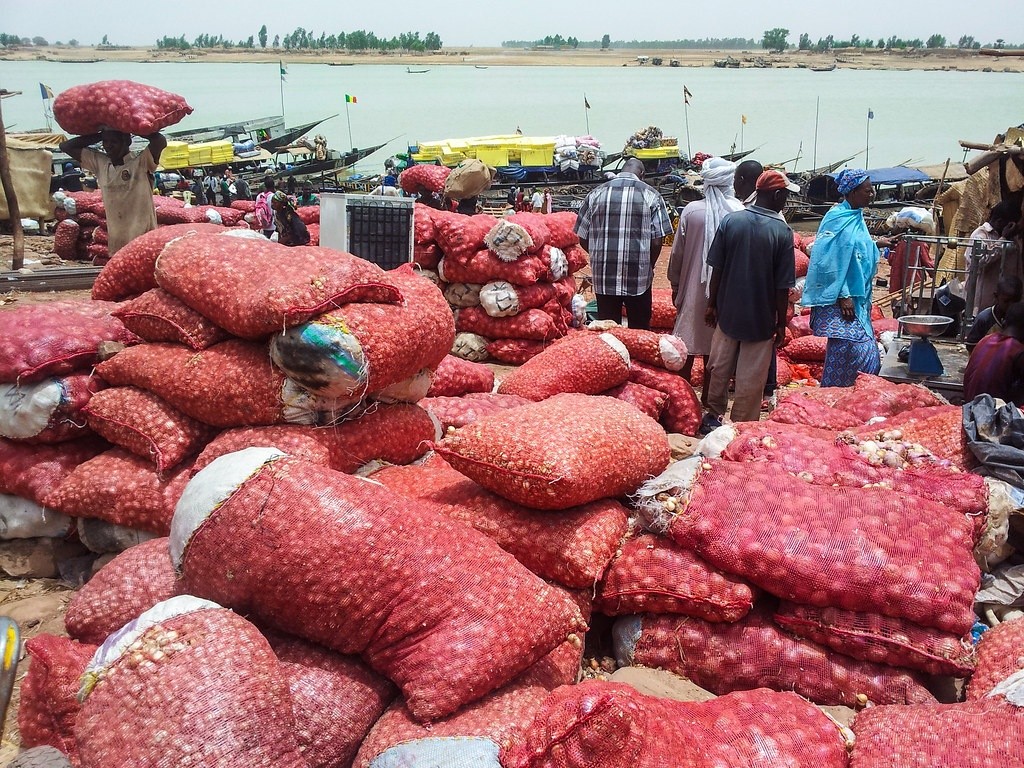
[700,413,722,435]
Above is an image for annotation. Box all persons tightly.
[255,176,320,247]
[368,176,484,217]
[155,166,251,206]
[801,167,908,388]
[963,200,1024,408]
[507,186,551,214]
[667,156,800,436]
[59,129,167,258]
[572,159,674,331]
[888,222,935,319]
[60,163,85,192]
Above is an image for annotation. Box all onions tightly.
[398,164,452,194]
[0,189,1024,768]
[52,80,192,135]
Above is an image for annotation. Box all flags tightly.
[40,82,54,99]
[868,111,874,119]
[346,94,357,103]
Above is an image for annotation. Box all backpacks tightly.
[255,191,272,229]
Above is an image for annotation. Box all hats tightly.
[66,163,72,169]
[756,170,800,193]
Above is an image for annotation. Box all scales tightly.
[898,315,954,377]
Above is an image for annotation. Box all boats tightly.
[45,57,107,63]
[325,62,355,66]
[404,69,431,73]
[808,65,838,72]
[152,114,971,219]
